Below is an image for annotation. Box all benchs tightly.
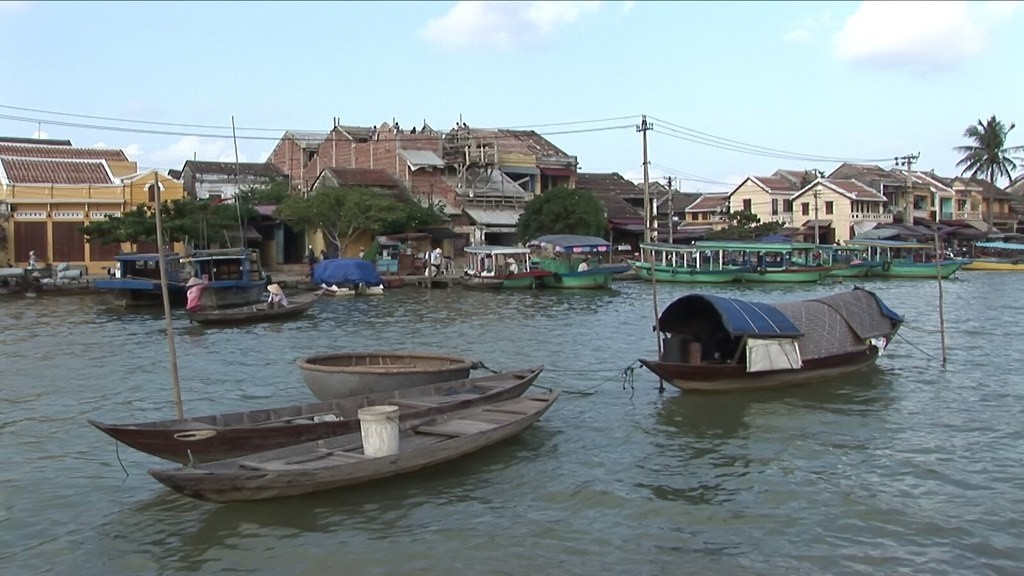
[391,396,465,409]
[416,420,495,435]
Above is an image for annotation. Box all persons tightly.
[578,259,588,272]
[186,278,211,312]
[29,250,38,269]
[306,245,316,266]
[266,283,286,308]
[360,246,365,259]
[431,247,442,277]
[506,257,518,274]
[321,250,329,260]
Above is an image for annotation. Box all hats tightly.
[435,247,442,254]
[267,283,282,295]
[186,277,204,287]
[507,257,517,264]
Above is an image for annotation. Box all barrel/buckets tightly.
[358,406,400,457]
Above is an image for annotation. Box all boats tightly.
[525,234,632,289]
[146,387,565,504]
[179,116,268,308]
[183,288,327,325]
[622,242,744,284]
[693,235,965,284]
[636,253,906,392]
[960,241,1024,271]
[85,171,547,467]
[310,257,385,296]
[464,244,553,290]
[94,250,191,307]
[458,275,504,290]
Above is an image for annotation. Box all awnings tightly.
[778,219,831,236]
